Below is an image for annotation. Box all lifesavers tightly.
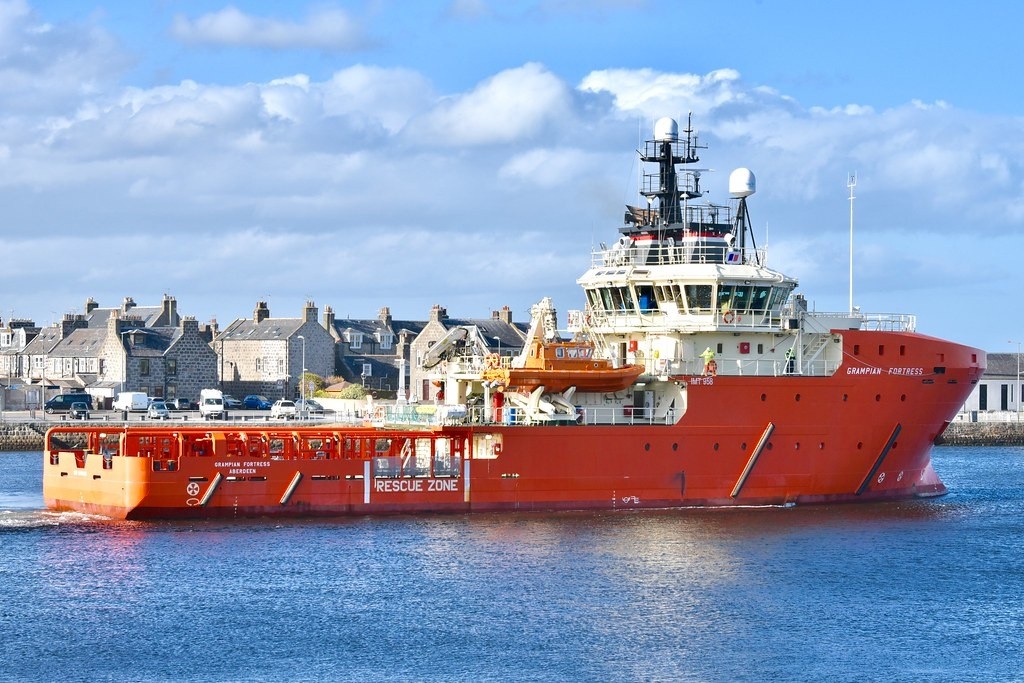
[706,359,717,373]
[723,311,734,323]
[587,316,595,327]
[492,353,500,367]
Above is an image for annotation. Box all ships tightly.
[40,107,990,517]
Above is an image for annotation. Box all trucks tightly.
[44,393,91,414]
[199,388,225,419]
[111,392,148,412]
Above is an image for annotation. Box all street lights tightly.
[360,371,367,387]
[297,335,306,411]
[492,335,501,355]
[41,333,48,421]
[220,338,224,386]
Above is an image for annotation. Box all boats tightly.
[478,296,647,395]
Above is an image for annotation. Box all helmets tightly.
[707,347,710,351]
[790,347,792,350]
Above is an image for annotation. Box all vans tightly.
[148,396,199,410]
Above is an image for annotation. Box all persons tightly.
[699,347,715,376]
[435,390,444,400]
[785,349,796,375]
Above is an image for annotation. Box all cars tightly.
[295,399,324,414]
[148,403,169,420]
[270,397,296,419]
[68,402,90,419]
[222,395,242,409]
[243,395,274,410]
